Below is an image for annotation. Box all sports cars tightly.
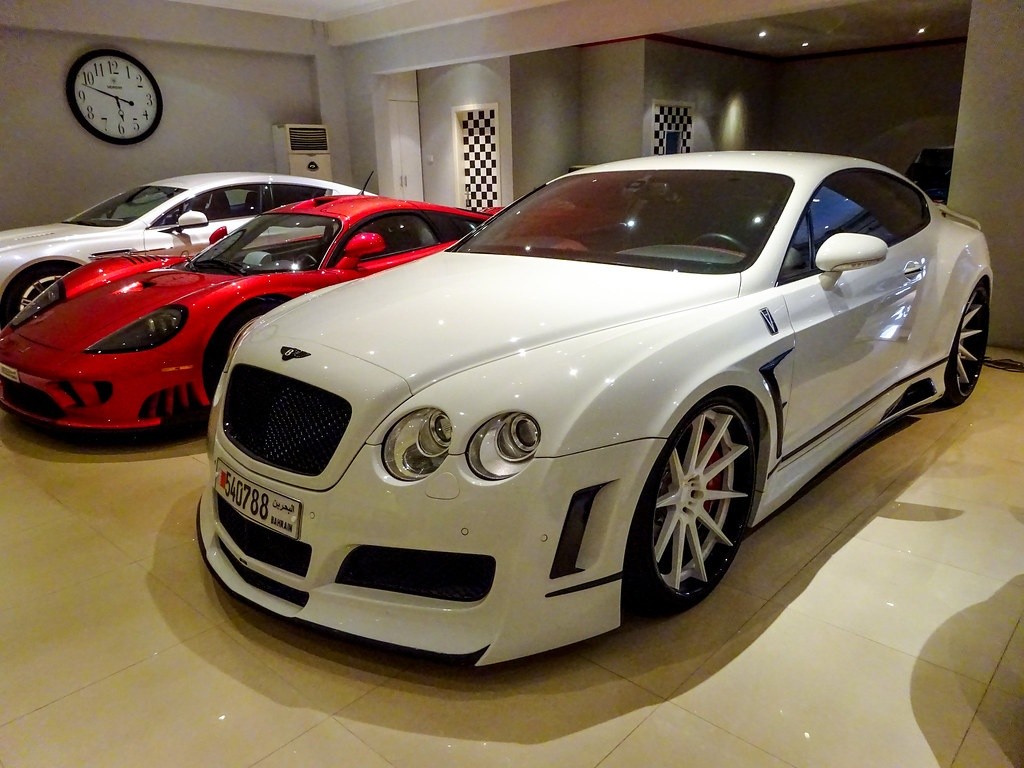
[0,195,505,429]
[194,150,996,666]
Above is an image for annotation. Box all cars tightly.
[0,172,387,329]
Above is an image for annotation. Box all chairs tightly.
[242,192,258,214]
[202,191,231,220]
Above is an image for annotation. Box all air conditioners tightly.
[271,124,334,182]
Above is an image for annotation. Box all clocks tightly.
[65,48,163,145]
